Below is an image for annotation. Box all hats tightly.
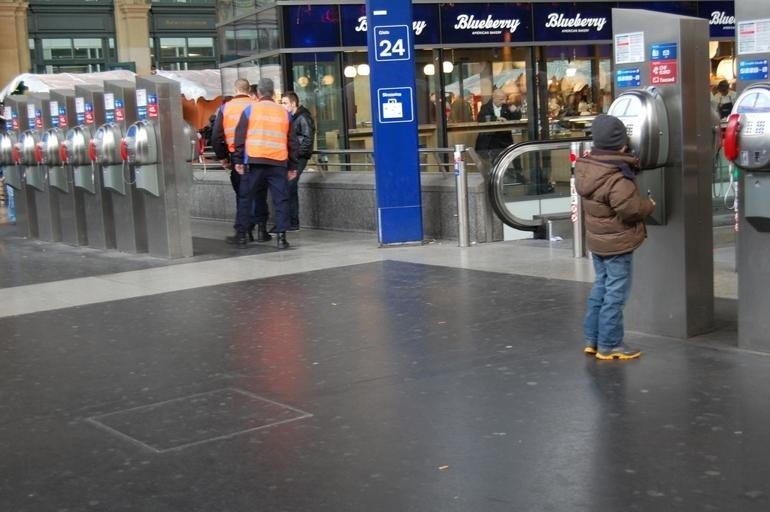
[591,113,630,153]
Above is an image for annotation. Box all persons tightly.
[449,88,473,121]
[199,76,315,250]
[572,112,657,360]
[714,79,736,121]
[474,89,528,185]
[429,93,438,122]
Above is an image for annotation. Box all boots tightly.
[257,229,272,243]
[226,231,246,249]
[244,230,254,242]
[276,232,290,249]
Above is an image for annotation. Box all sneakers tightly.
[583,339,598,354]
[284,222,300,233]
[515,176,529,186]
[595,340,642,362]
[267,226,287,236]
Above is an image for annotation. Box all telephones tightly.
[1,118,205,167]
[606,86,670,171]
[723,83,770,172]
